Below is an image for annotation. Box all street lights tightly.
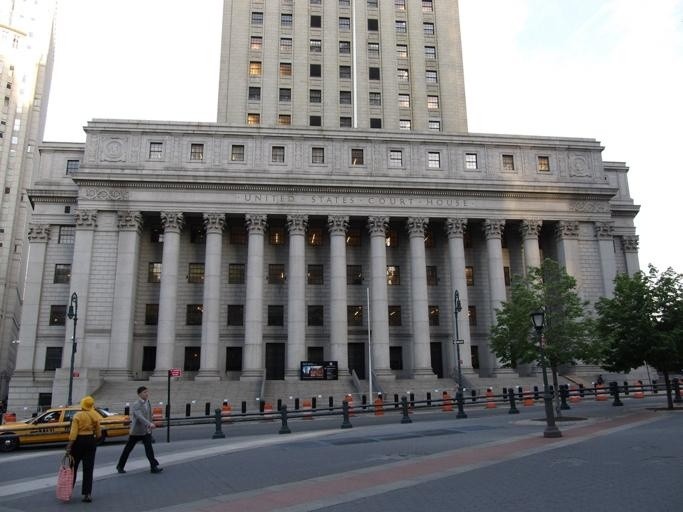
[529,311,562,438]
[66,292,78,407]
[453,290,467,419]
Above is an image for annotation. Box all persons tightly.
[116,386,163,473]
[46,411,60,423]
[65,396,101,502]
[597,374,603,384]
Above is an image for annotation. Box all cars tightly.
[1,405,130,451]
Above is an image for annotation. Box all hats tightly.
[80,396,95,411]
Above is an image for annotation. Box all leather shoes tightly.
[151,468,164,473]
[116,466,126,473]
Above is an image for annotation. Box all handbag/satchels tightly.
[56,465,74,502]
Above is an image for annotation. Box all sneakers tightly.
[81,496,92,503]
